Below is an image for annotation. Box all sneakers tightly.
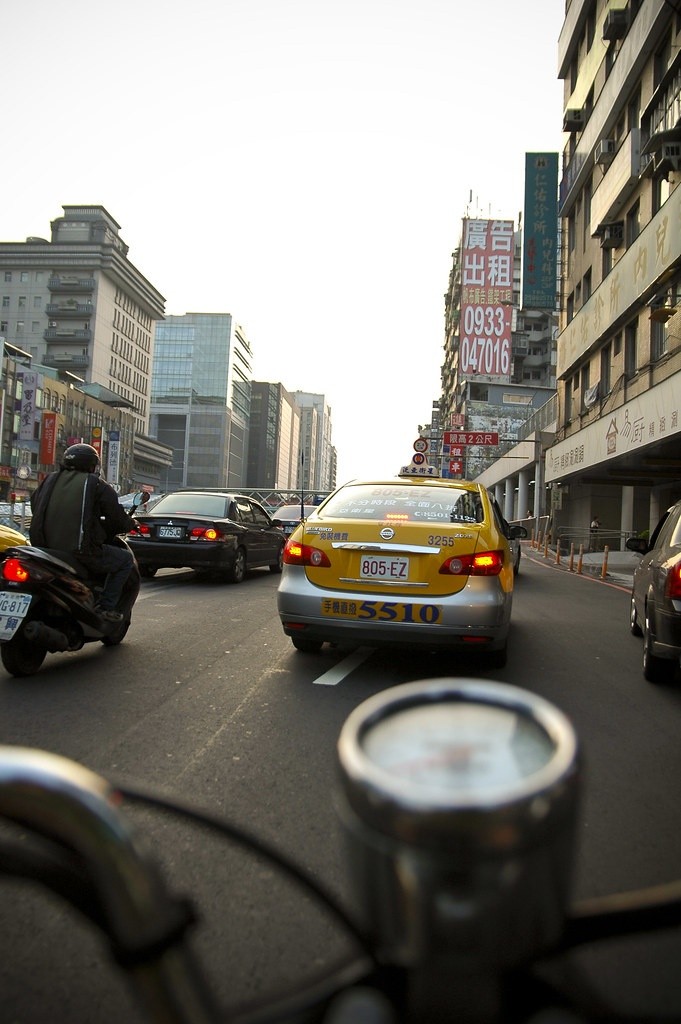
[94,604,123,622]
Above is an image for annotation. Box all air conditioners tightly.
[562,108,582,131]
[601,8,626,40]
[54,407,60,412]
[599,226,622,248]
[594,139,614,162]
[49,322,58,327]
[652,141,681,174]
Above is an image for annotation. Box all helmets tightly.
[63,444,101,469]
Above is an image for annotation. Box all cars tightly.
[117,493,166,520]
[269,504,321,538]
[125,490,287,583]
[276,451,527,669]
[622,499,681,684]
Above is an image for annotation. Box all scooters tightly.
[0,491,151,680]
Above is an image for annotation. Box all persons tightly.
[589,516,599,553]
[29,443,140,624]
